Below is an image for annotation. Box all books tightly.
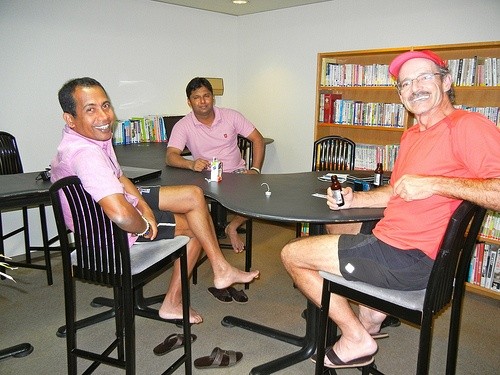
[320,141,401,171]
[479,209,500,241]
[467,242,500,293]
[447,55,500,87]
[453,104,500,127]
[321,57,398,86]
[111,114,168,145]
[319,93,404,128]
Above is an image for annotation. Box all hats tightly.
[389,50,445,77]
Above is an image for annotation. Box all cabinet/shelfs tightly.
[310,39,500,302]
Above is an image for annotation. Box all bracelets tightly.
[135,216,151,237]
[250,166,261,174]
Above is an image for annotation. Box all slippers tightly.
[208,286,234,303]
[227,287,248,304]
[310,346,375,368]
[337,333,388,338]
[194,347,243,369]
[153,333,197,356]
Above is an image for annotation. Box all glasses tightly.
[397,73,444,90]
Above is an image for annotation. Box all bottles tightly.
[373,163,383,188]
[330,175,344,207]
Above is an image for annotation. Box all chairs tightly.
[315,199,488,375]
[311,135,357,173]
[49,176,194,375]
[0,130,75,287]
[191,132,254,290]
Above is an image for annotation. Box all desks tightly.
[163,114,274,240]
[0,144,398,375]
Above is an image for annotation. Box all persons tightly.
[51,77,259,323]
[166,77,266,253]
[280,49,500,369]
